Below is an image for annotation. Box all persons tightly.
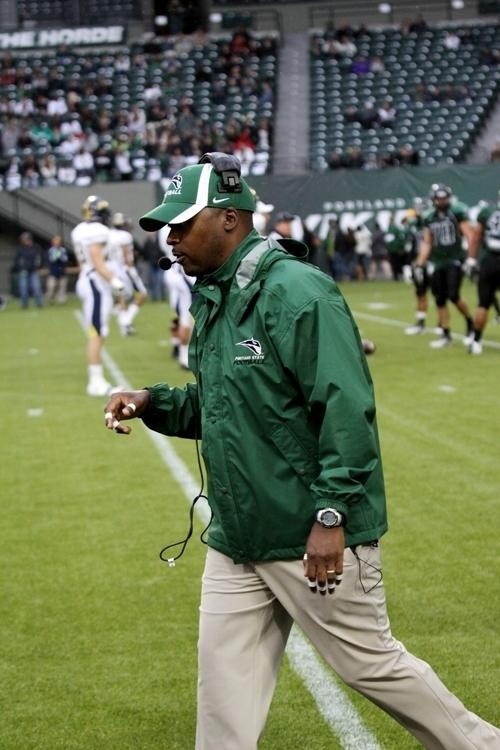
[106,154,499,750]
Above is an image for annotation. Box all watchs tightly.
[315,507,343,528]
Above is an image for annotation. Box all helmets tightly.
[82,196,110,222]
[430,183,452,200]
[113,211,133,227]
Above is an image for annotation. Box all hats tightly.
[256,201,274,213]
[138,163,254,231]
[273,211,293,222]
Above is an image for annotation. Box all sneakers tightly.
[87,381,125,396]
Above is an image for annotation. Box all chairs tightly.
[309,24,500,172]
[0,42,276,183]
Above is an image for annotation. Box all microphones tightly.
[158,256,182,272]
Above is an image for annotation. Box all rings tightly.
[326,570,337,574]
